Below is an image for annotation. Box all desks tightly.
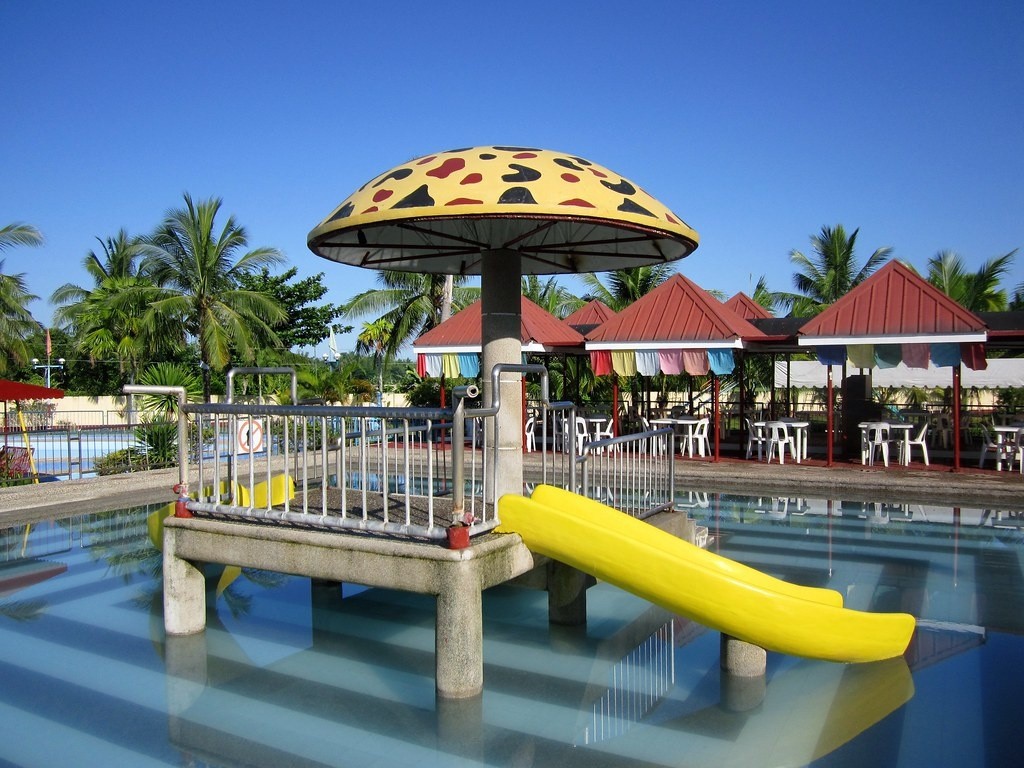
[560,418,607,455]
[991,426,1024,471]
[858,422,913,467]
[753,420,809,463]
[649,418,700,459]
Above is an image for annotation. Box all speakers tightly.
[842,375,873,459]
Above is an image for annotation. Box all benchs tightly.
[0,445,34,478]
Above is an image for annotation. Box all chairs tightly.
[525,413,1023,473]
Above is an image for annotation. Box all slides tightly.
[569,654,914,768]
[495,484,917,665]
[146,473,295,552]
